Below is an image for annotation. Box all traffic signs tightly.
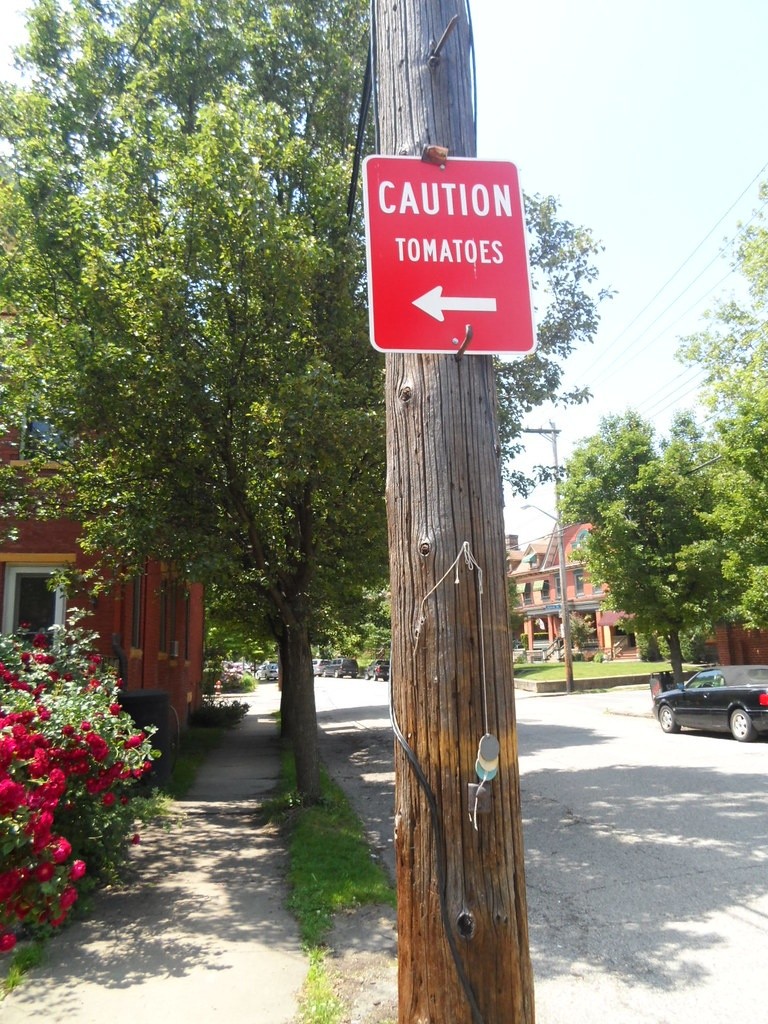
[363,154,536,356]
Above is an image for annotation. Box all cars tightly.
[651,665,768,743]
[255,654,360,681]
[364,659,389,680]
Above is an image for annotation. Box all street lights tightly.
[521,504,575,694]
